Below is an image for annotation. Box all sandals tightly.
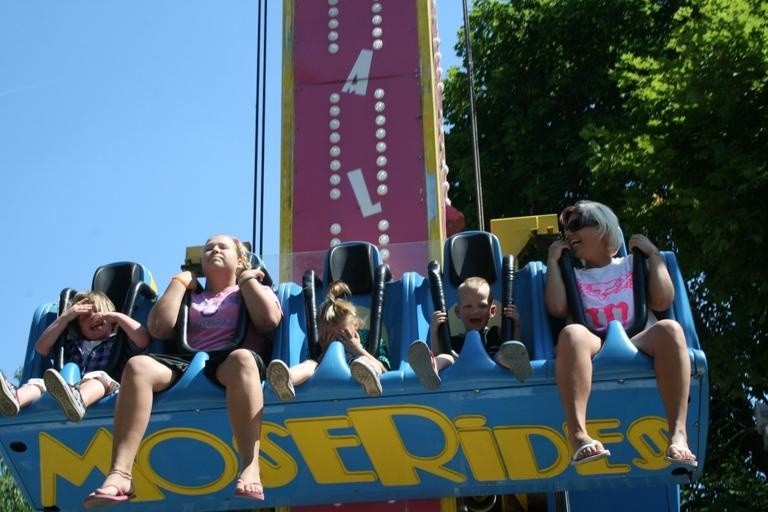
[83,469,137,509]
[233,478,265,502]
[406,341,442,390]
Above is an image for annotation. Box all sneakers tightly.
[0,372,20,416]
[499,340,532,381]
[43,369,85,422]
[267,359,296,401]
[350,359,382,397]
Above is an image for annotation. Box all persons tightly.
[80,232,284,508]
[265,277,390,402]
[0,287,150,425]
[543,197,702,467]
[407,275,532,392]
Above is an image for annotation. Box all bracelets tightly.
[238,275,258,288]
[170,276,189,290]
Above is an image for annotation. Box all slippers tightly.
[569,440,611,467]
[663,445,698,468]
